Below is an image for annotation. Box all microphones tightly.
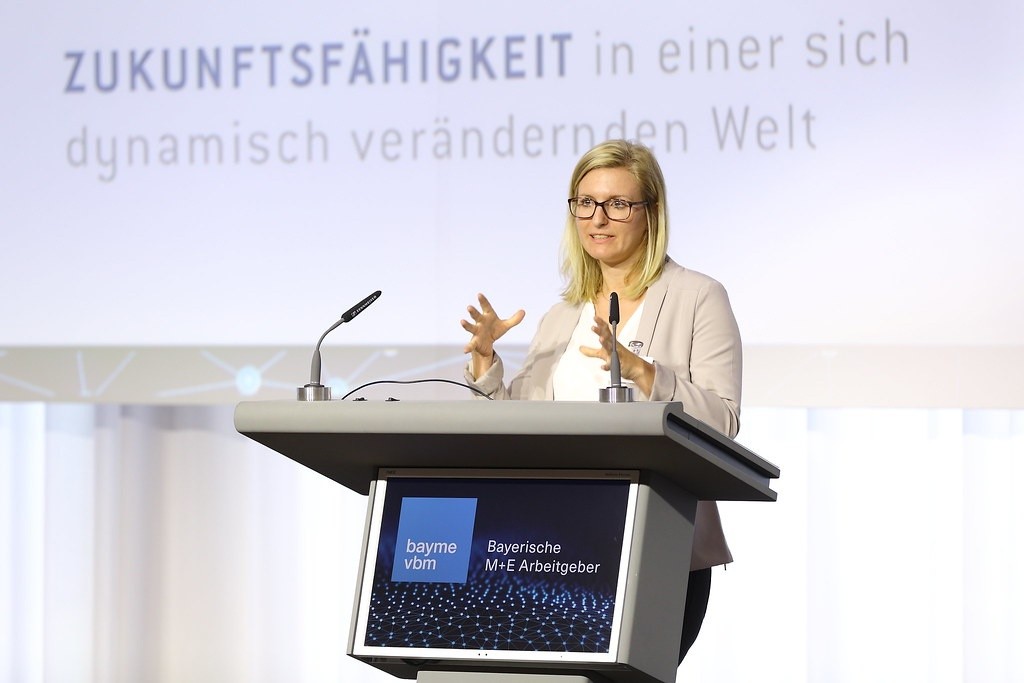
[296,291,382,401]
[599,292,632,402]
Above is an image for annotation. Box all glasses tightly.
[568,195,648,222]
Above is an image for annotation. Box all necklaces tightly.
[601,287,623,302]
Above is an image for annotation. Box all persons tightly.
[461,140,745,667]
